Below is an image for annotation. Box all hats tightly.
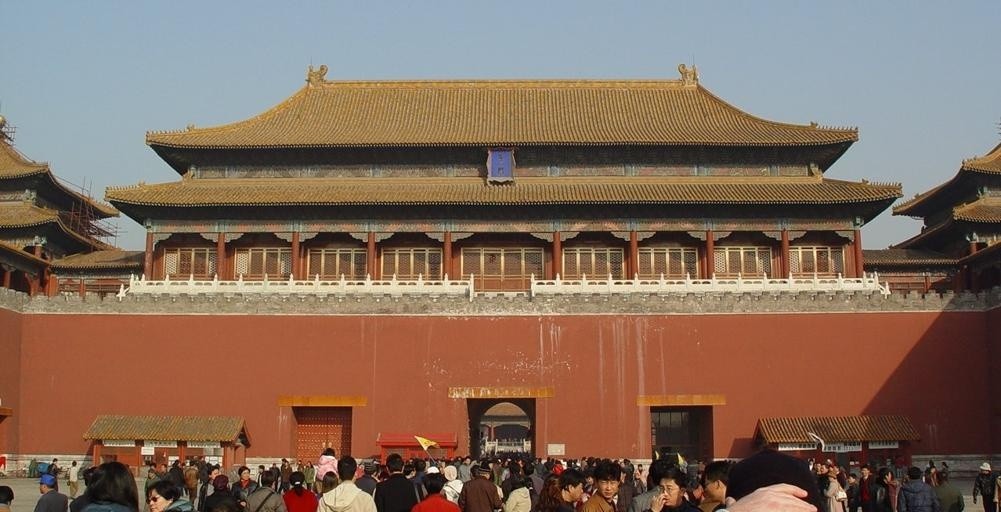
[828,471,836,479]
[980,463,992,471]
[443,465,457,481]
[40,475,55,486]
[479,460,491,473]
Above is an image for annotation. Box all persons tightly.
[0,433,1000,512]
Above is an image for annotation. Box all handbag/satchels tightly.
[836,487,848,502]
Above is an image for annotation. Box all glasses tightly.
[146,495,160,504]
